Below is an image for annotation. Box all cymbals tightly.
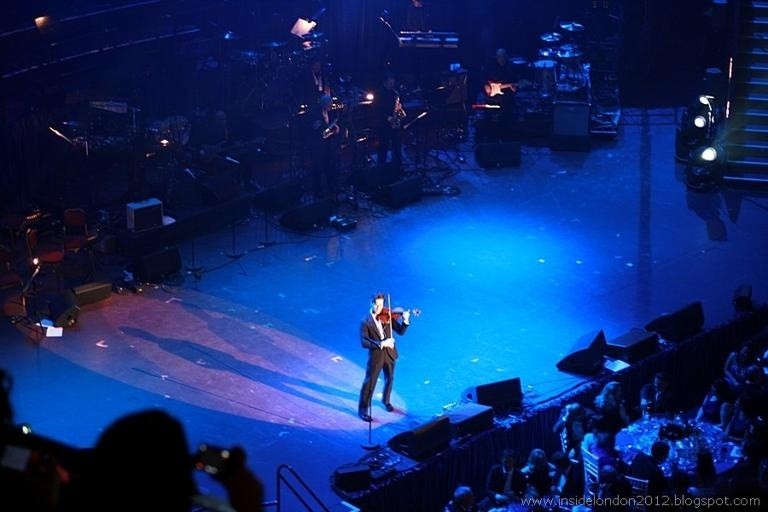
[560,21,585,33]
[560,43,583,51]
[541,32,562,43]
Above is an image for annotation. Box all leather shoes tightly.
[361,415,371,421]
[386,404,393,411]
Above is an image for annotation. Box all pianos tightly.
[399,31,459,49]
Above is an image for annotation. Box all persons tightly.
[310,97,343,190]
[209,110,234,143]
[355,288,411,421]
[370,72,404,173]
[2,332,768,510]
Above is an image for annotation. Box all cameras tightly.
[193,443,231,476]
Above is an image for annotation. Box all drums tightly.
[540,47,557,60]
[556,65,585,93]
[558,52,584,65]
[528,60,560,91]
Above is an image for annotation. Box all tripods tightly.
[13,234,52,334]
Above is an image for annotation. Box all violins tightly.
[377,307,420,325]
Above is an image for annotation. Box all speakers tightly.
[555,329,607,376]
[644,300,705,343]
[387,416,450,461]
[459,377,523,414]
[335,465,371,488]
[371,175,424,209]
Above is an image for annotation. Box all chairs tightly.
[579,446,600,495]
[0,208,97,307]
[625,476,649,512]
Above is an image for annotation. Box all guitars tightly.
[484,82,524,99]
[200,136,267,166]
